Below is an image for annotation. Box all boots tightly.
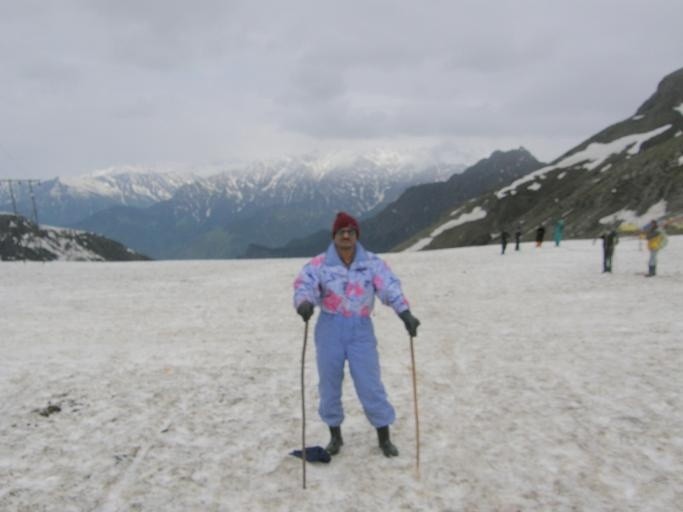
[326,426,342,454]
[378,426,397,456]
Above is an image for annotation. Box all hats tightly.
[333,212,359,238]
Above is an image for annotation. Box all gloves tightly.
[299,301,313,321]
[400,310,419,335]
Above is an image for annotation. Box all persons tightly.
[599,222,619,273]
[294,209,422,458]
[499,229,510,255]
[553,216,567,247]
[535,221,546,249]
[513,226,522,250]
[639,219,669,276]
